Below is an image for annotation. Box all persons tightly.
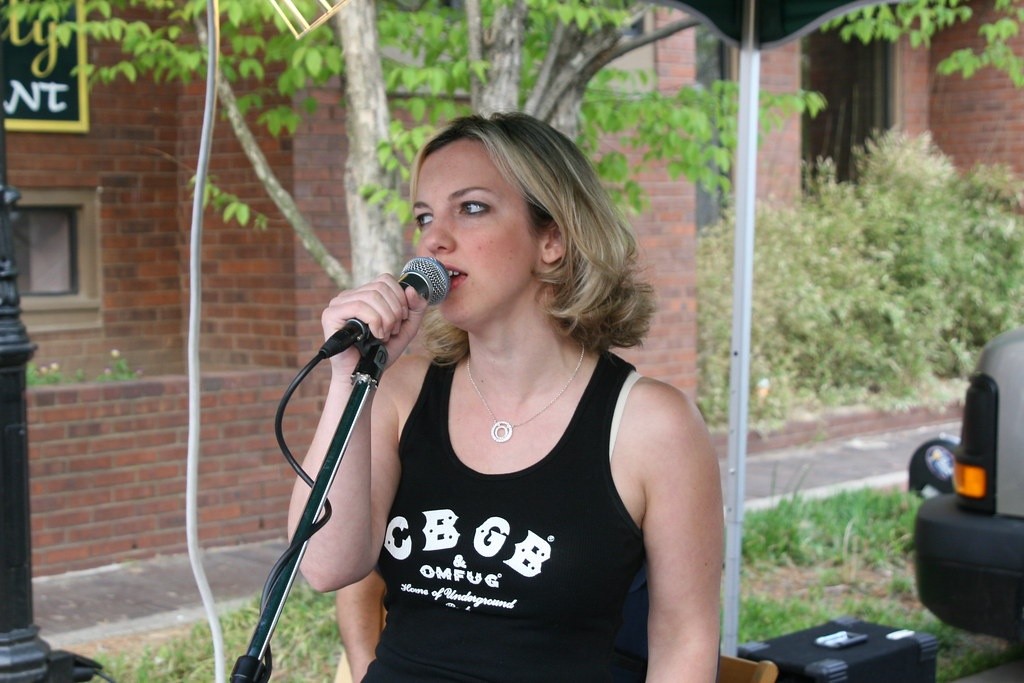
[285,111,721,683]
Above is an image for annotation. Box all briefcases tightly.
[737,615,937,683]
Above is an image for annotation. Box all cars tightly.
[910,327,1024,643]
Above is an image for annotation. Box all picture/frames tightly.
[0,0,91,133]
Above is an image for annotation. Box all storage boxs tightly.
[739,614,937,683]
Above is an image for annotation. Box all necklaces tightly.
[464,339,587,444]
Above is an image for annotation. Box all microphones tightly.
[320,255,452,360]
